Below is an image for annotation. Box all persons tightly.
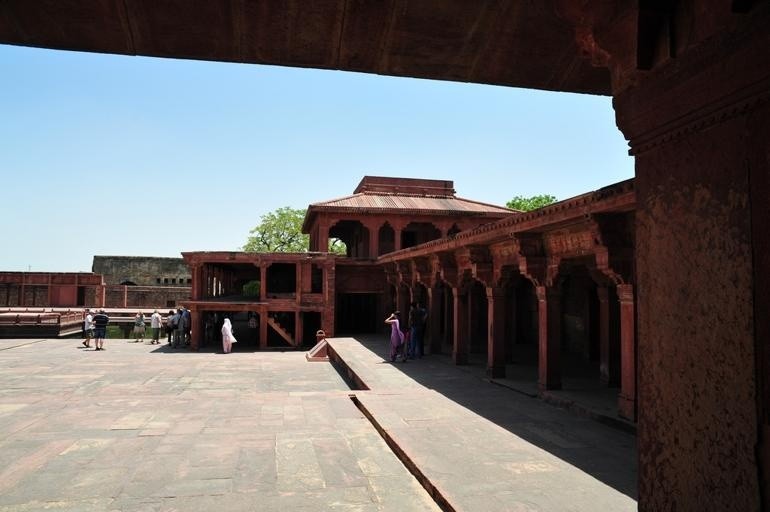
[83,311,97,348]
[220,318,238,354]
[134,310,146,342]
[92,309,109,350]
[382,299,429,362]
[150,305,192,349]
